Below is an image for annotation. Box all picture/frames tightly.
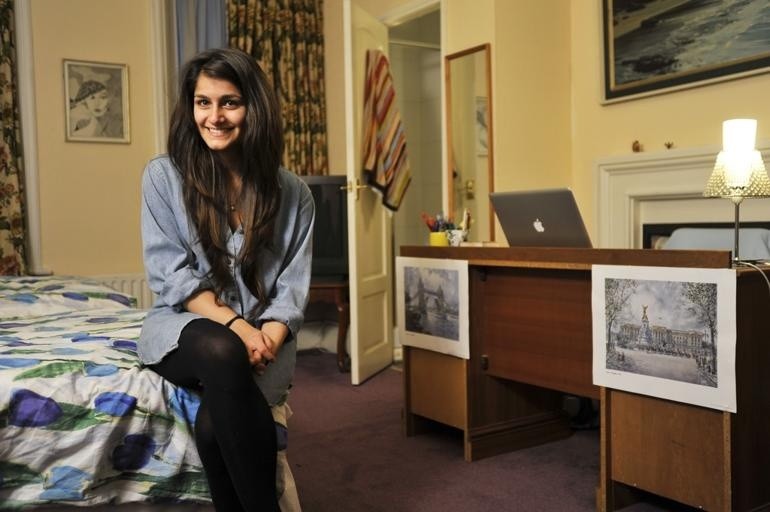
[63,58,131,144]
[600,0,770,104]
[476,97,488,155]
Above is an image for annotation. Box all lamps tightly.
[702,119,770,265]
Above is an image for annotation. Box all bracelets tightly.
[225,315,244,328]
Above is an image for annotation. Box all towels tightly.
[363,48,411,213]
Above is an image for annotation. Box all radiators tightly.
[101,274,156,311]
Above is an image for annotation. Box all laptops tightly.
[489,187,592,248]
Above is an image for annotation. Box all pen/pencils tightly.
[421,206,473,231]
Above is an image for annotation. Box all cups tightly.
[428,231,450,247]
[444,228,470,246]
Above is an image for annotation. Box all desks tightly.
[305,282,351,375]
[401,245,770,512]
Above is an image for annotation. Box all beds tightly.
[0,275,138,317]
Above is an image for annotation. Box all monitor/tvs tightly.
[296,175,349,284]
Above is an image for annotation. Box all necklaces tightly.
[229,195,240,213]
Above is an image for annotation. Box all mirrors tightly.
[445,44,495,244]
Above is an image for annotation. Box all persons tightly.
[76,80,112,118]
[137,51,318,511]
